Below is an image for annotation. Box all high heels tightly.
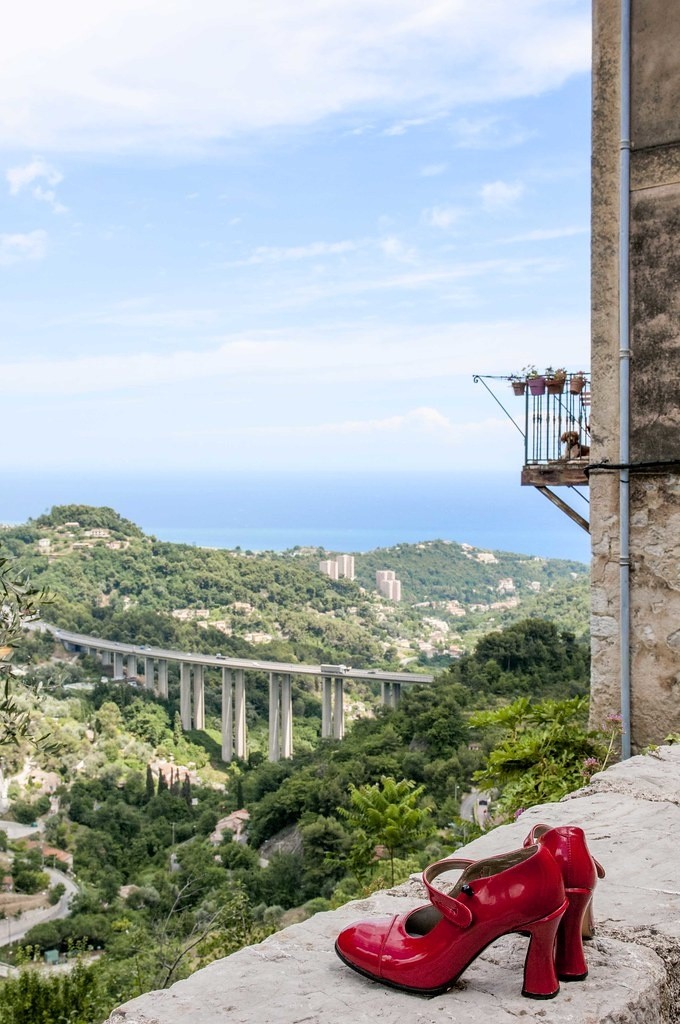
[525,822,606,983]
[334,841,568,999]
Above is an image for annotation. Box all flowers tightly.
[570,370,587,384]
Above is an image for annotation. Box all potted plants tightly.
[544,367,567,394]
[521,364,546,396]
[510,373,527,396]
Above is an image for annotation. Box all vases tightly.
[570,379,585,395]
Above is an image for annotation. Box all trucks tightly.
[321,664,351,675]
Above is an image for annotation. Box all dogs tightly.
[561,431,589,462]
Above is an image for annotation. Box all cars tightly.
[369,671,376,674]
